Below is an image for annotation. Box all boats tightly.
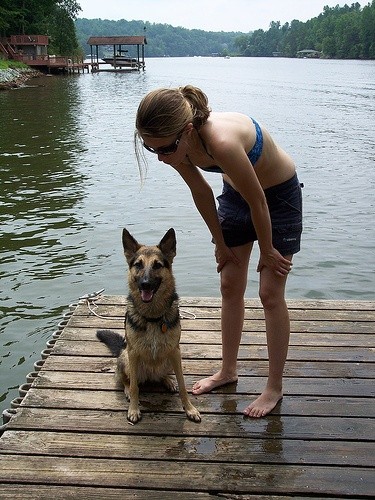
[102,50,138,65]
[224,56,230,59]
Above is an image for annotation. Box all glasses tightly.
[142,128,186,154]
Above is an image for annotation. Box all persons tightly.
[134,84,308,419]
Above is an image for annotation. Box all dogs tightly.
[96,227,202,424]
